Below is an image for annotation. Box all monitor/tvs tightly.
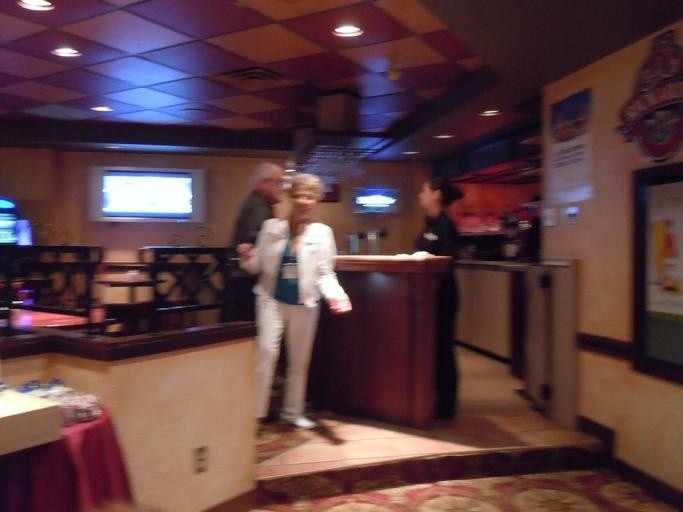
[86,167,208,223]
[352,187,401,213]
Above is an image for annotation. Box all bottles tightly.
[658,219,677,289]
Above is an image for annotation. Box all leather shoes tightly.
[278,413,318,428]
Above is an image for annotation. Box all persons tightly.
[215,159,287,324]
[409,175,467,423]
[502,193,545,383]
[233,170,355,432]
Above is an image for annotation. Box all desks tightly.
[0,383,134,512]
